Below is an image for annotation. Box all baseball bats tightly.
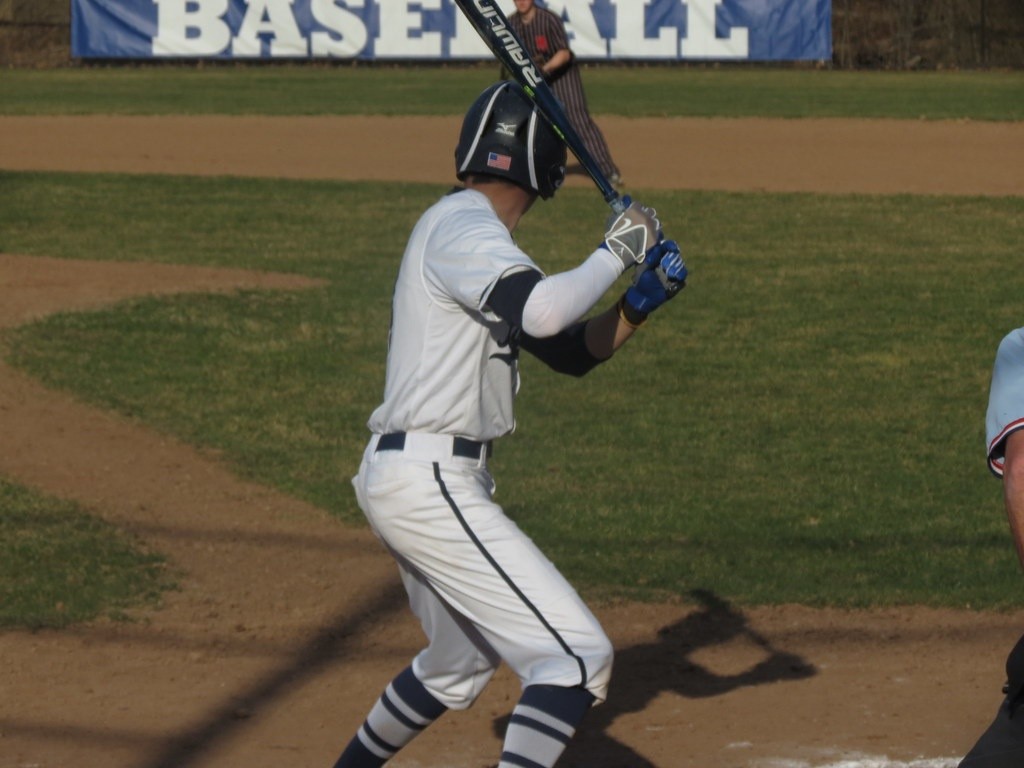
[453,0,685,300]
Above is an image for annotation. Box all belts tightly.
[374,432,493,460]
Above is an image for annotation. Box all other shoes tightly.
[606,167,621,190]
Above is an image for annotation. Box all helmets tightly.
[454,80,568,201]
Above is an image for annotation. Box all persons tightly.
[336,83,687,767]
[501,0,622,189]
[958,329,1024,768]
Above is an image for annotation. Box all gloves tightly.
[599,194,664,272]
[621,240,687,325]
